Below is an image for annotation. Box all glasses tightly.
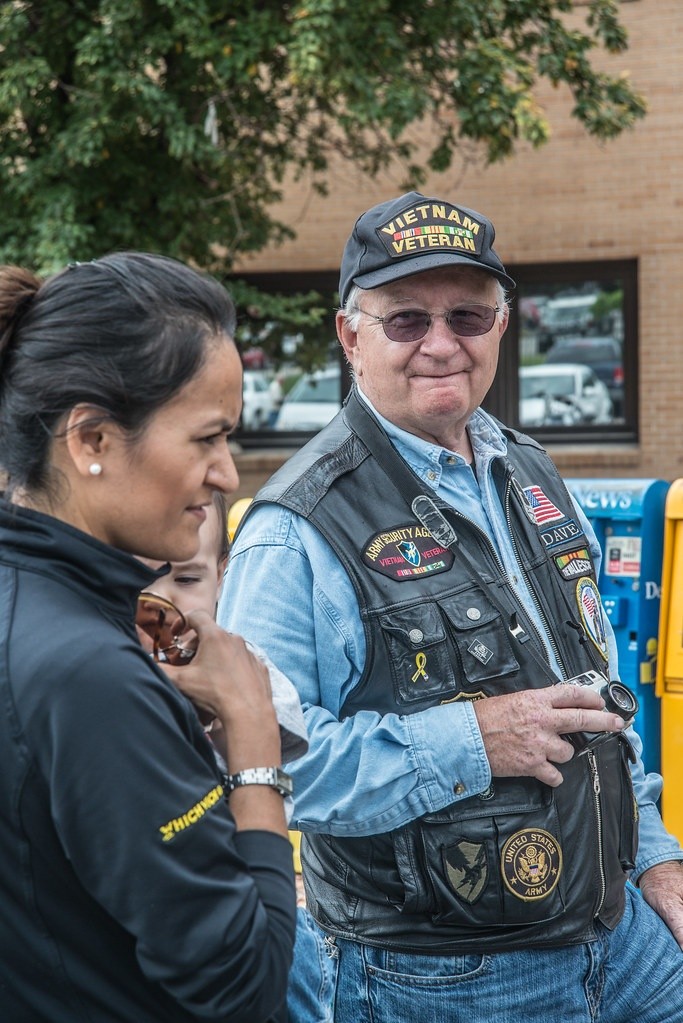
[346,304,500,344]
[132,591,218,726]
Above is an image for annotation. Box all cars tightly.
[519,295,624,425]
[242,336,340,430]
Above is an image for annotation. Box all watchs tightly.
[221,764,294,800]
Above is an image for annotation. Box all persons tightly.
[204,188,682,1023]
[2,253,299,1023]
[116,498,338,1022]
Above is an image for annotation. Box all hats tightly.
[339,191,516,307]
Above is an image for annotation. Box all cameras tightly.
[555,670,640,761]
